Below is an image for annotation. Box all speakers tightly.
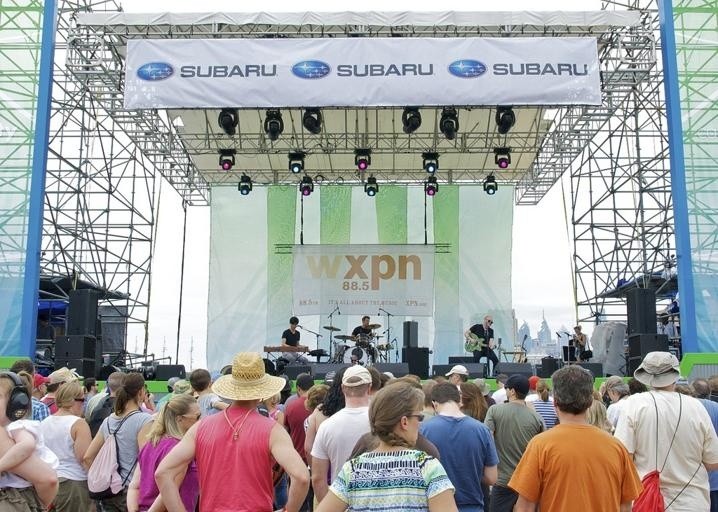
[285,365,311,381]
[375,364,409,378]
[451,363,487,379]
[402,347,428,381]
[56,336,96,383]
[311,362,352,381]
[494,362,532,379]
[627,286,658,333]
[66,288,98,336]
[156,365,186,381]
[627,333,668,377]
[403,321,418,350]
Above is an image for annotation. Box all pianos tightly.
[263,345,309,352]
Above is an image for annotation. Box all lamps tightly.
[364,177,379,196]
[287,152,305,173]
[483,176,498,195]
[401,106,423,135]
[218,149,235,169]
[424,177,438,195]
[353,148,373,170]
[421,152,441,173]
[301,108,322,134]
[218,109,239,136]
[439,107,459,139]
[493,148,511,169]
[299,176,317,196]
[264,109,283,140]
[237,176,253,195]
[495,107,515,133]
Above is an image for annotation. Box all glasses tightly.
[408,414,424,421]
[182,413,202,420]
[74,398,85,402]
[94,384,99,386]
[487,319,493,324]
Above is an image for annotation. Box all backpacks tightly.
[631,390,702,512]
[88,410,141,499]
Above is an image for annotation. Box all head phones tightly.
[3,370,30,420]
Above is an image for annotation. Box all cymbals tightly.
[323,326,341,331]
[367,324,381,329]
[374,335,384,338]
[334,335,353,339]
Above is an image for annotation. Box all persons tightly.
[82,373,154,512]
[313,382,459,512]
[473,379,495,405]
[190,369,229,416]
[526,375,553,402]
[0,425,59,512]
[671,301,679,318]
[445,364,469,392]
[351,316,371,346]
[311,365,372,505]
[606,375,631,427]
[464,316,499,377]
[492,377,508,403]
[532,381,558,428]
[126,395,201,512]
[657,313,679,342]
[305,366,354,467]
[692,378,718,512]
[83,378,99,417]
[32,374,51,420]
[154,352,310,512]
[460,384,488,420]
[89,372,126,438]
[10,359,50,421]
[40,370,72,414]
[614,351,718,512]
[41,383,92,512]
[0,371,60,490]
[284,374,316,466]
[508,365,644,512]
[281,317,308,365]
[418,382,500,512]
[573,325,586,362]
[483,376,547,512]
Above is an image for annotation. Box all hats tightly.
[474,378,490,396]
[325,371,337,382]
[529,376,539,391]
[58,367,77,377]
[211,352,286,402]
[445,365,468,376]
[33,374,50,388]
[634,351,681,388]
[342,365,372,386]
[384,372,396,379]
[47,368,71,386]
[72,372,84,380]
[168,376,180,389]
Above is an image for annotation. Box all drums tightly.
[355,333,370,348]
[344,346,367,367]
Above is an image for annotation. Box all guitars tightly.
[465,333,497,353]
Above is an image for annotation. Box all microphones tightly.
[382,328,389,334]
[486,324,491,328]
[378,308,381,316]
[337,305,340,315]
[556,332,561,338]
[564,331,572,336]
[297,324,301,327]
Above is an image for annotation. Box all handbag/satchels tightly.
[580,335,593,360]
